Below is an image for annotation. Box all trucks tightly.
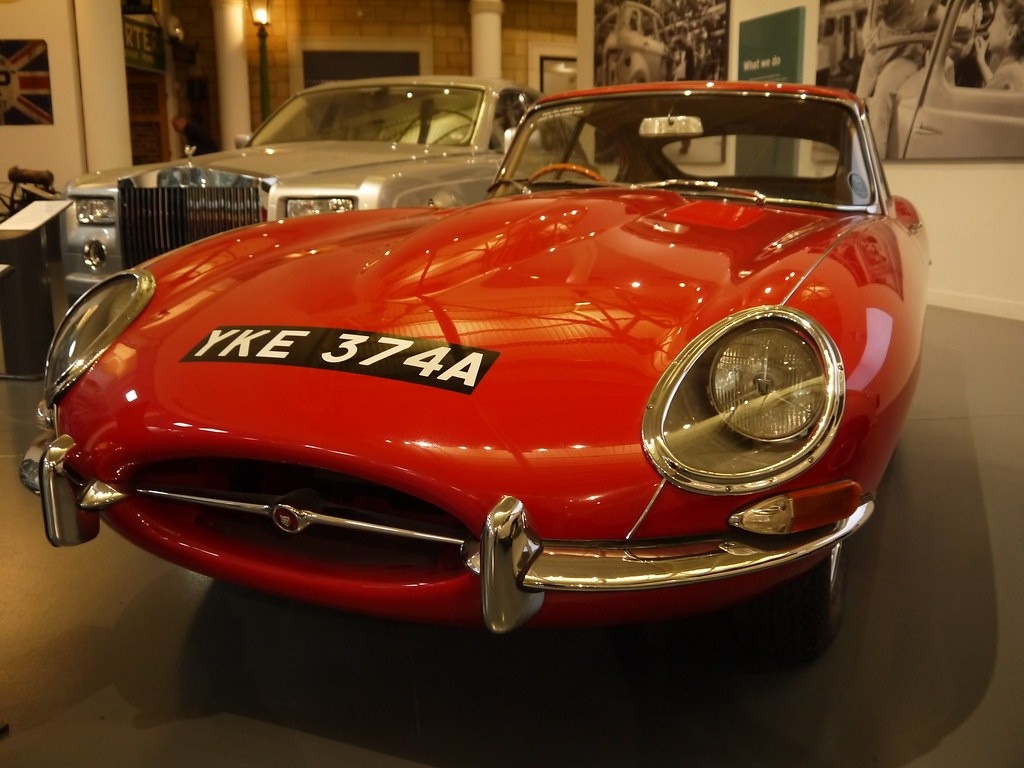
[817,1,870,84]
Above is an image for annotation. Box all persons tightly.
[172,117,220,156]
[854,0,1024,162]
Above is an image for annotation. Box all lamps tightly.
[247,0,271,27]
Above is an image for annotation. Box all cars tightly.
[603,1,671,84]
[64,76,601,302]
[868,0,1024,157]
[19,79,929,659]
